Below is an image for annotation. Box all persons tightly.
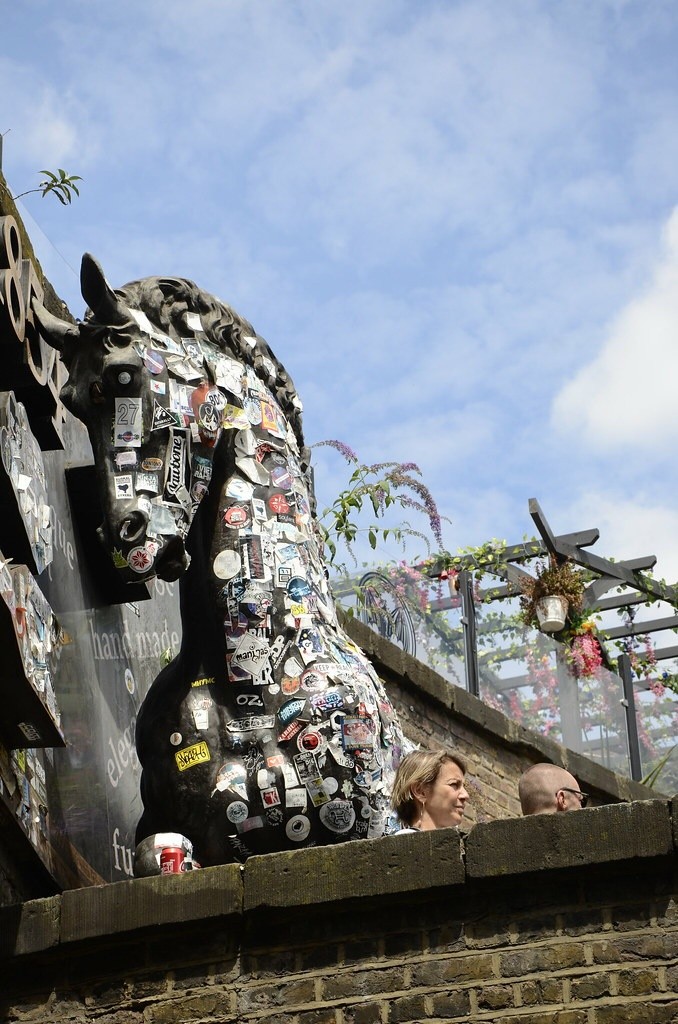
[392,750,470,837]
[518,763,582,817]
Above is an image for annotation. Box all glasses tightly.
[555,787,589,808]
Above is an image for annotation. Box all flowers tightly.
[310,440,451,576]
[366,535,678,797]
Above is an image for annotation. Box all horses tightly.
[29,251,424,868]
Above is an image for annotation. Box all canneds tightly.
[159,847,185,875]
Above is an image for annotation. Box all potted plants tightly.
[516,553,591,632]
